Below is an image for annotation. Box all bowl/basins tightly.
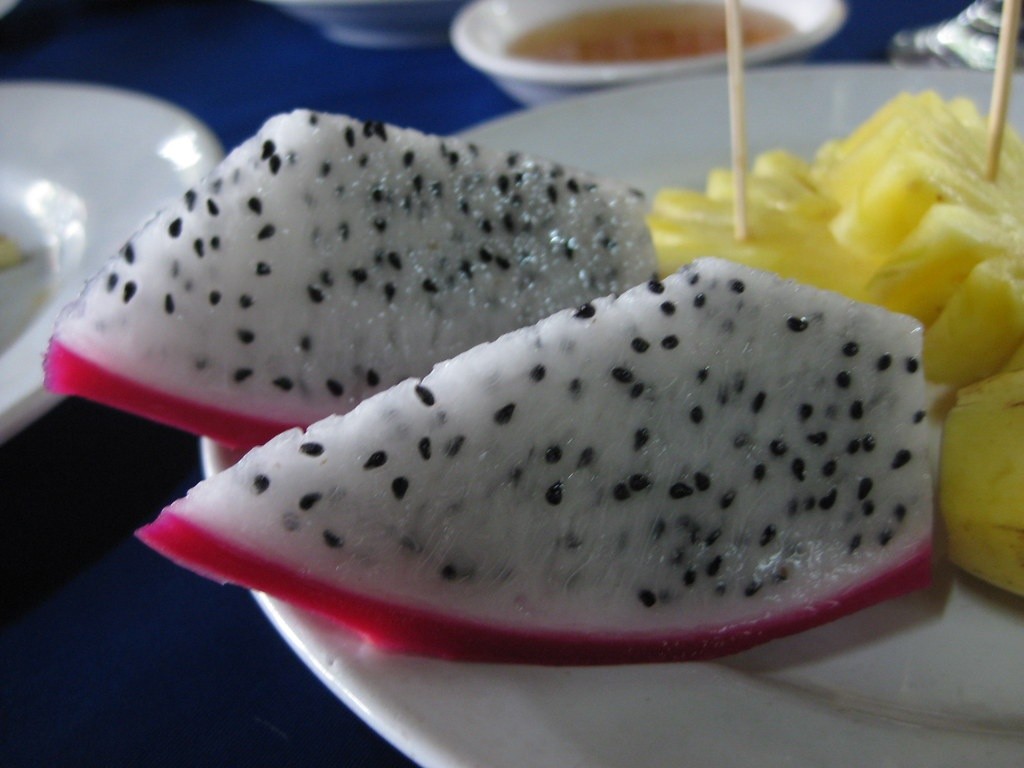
[449,0,848,111]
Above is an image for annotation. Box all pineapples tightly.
[640,88,1024,603]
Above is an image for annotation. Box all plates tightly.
[199,60,1024,768]
[0,77,225,447]
[270,0,475,49]
